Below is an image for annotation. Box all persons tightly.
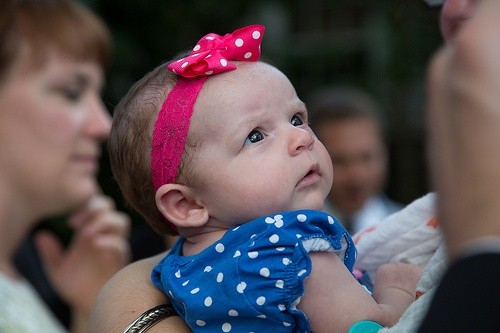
[109,24,424,333]
[0,0,500,333]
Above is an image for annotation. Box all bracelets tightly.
[121,303,179,333]
